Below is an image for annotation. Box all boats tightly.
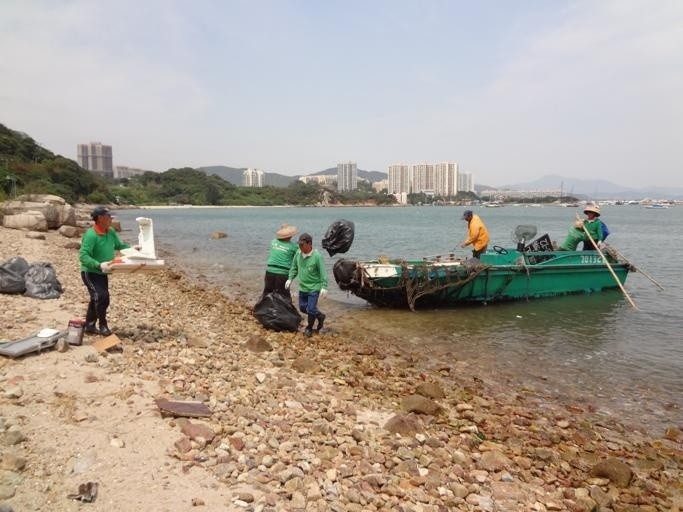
[332,245,629,305]
[484,200,677,209]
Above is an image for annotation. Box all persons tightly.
[78,206,141,337]
[574,206,602,251]
[459,210,489,259]
[263,224,298,306]
[284,233,327,333]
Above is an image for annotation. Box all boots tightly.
[86,302,100,333]
[315,313,325,329]
[100,307,111,335]
[305,316,315,336]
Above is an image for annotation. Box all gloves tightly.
[285,280,290,290]
[100,261,114,274]
[320,289,328,299]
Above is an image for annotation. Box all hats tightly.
[276,224,297,239]
[297,233,312,244]
[584,205,600,217]
[91,206,116,219]
[461,210,472,220]
